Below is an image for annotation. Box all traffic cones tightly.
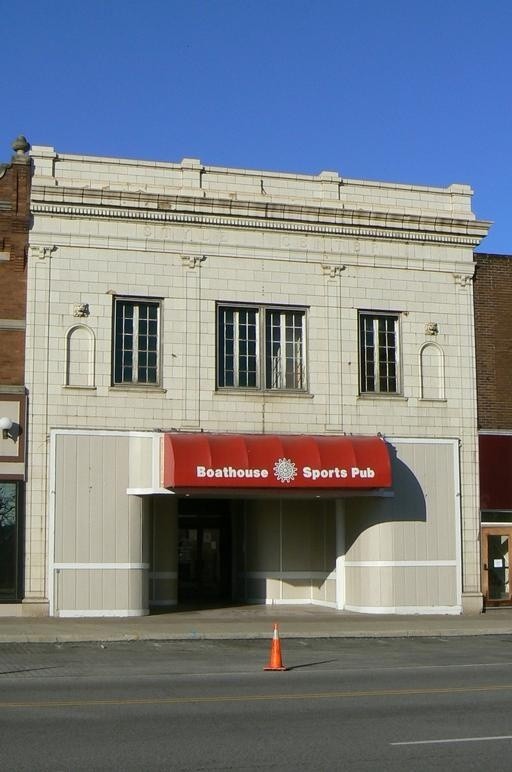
[264,624,287,669]
